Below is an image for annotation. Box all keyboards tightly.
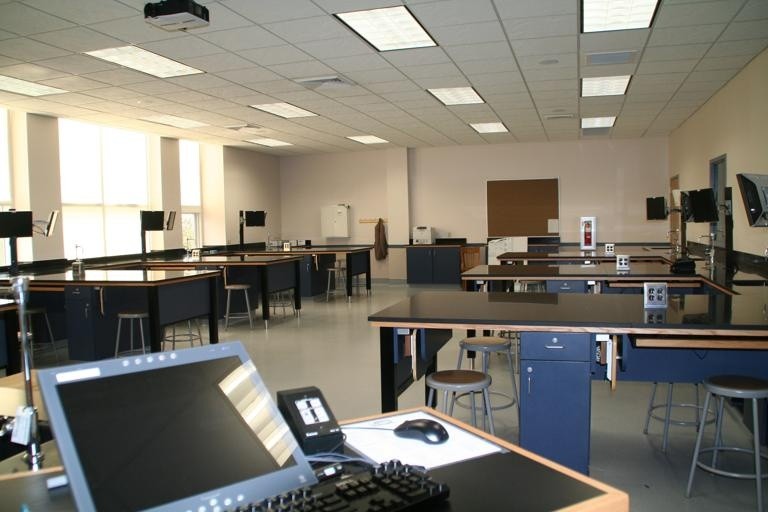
[225,459,450,512]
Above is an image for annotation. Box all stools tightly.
[426,336,520,437]
[162,319,203,351]
[114,311,151,359]
[643,374,768,510]
[224,284,254,330]
[18,308,59,370]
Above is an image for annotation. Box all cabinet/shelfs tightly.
[320,203,352,239]
[406,247,460,284]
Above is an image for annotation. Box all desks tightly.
[368,291,767,477]
[1,270,222,359]
[141,245,371,320]
[460,246,767,295]
[0,400,634,512]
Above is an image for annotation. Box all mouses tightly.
[396,418,448,444]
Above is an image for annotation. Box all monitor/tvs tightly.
[45,209,58,236]
[245,211,265,227]
[0,211,32,237]
[646,197,667,220]
[736,173,768,227]
[167,211,176,230]
[688,188,720,222]
[36,340,319,512]
[142,211,164,230]
[680,191,694,223]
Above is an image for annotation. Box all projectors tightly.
[144,0,209,32]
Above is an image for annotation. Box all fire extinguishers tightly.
[585,223,591,246]
